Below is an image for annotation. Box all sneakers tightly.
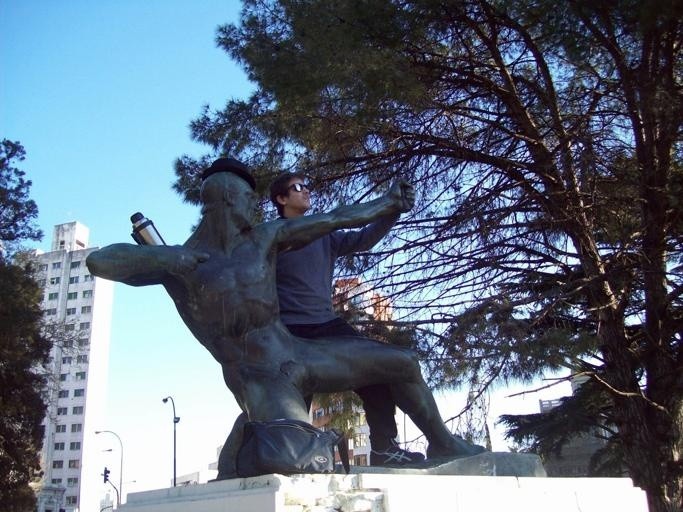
[370,445,425,466]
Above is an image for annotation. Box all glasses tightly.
[279,182,308,193]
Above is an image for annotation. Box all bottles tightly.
[130,212,168,247]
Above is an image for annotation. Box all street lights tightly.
[112,479,136,511]
[94,430,122,506]
[101,473,119,507]
[161,395,180,487]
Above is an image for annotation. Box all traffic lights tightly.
[103,466,110,483]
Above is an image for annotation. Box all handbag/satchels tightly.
[235,416,344,474]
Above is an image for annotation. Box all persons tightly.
[85,168,490,481]
[268,170,427,469]
